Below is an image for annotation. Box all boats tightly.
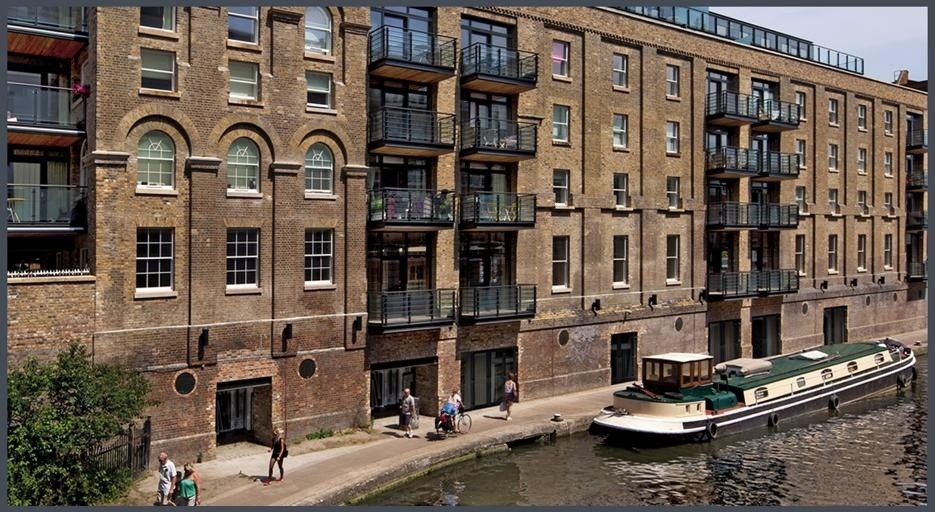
[579,318,920,447]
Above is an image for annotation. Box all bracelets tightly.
[279,456,281,458]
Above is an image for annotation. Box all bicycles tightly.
[435,404,475,438]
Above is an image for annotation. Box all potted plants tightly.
[906,171,928,186]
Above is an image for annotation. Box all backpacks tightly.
[272,438,289,459]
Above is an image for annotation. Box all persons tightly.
[263,427,286,483]
[441,386,465,434]
[157,452,202,506]
[72,76,90,103]
[373,188,454,221]
[503,372,517,420]
[399,387,416,438]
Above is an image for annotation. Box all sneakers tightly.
[403,432,414,440]
[504,415,513,421]
[262,478,285,486]
[448,429,460,434]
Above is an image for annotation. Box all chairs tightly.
[485,201,519,223]
[711,152,725,166]
[468,127,518,150]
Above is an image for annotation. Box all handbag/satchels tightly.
[499,400,508,412]
[511,391,519,403]
[398,413,405,426]
[410,416,419,429]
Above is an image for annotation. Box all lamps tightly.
[286,321,295,339]
[851,277,859,286]
[200,326,209,344]
[879,276,885,284]
[593,296,601,310]
[820,280,828,289]
[648,294,657,305]
[353,315,364,334]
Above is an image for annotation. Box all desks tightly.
[415,43,431,63]
